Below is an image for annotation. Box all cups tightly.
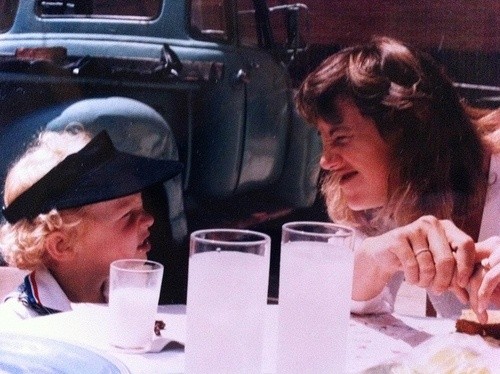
[184,229,271,374]
[277,221,353,374]
[107,259,164,354]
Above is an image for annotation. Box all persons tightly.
[0,97,185,324]
[296,37,500,323]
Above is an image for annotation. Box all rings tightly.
[415,246,430,257]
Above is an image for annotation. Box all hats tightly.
[1,129,184,225]
